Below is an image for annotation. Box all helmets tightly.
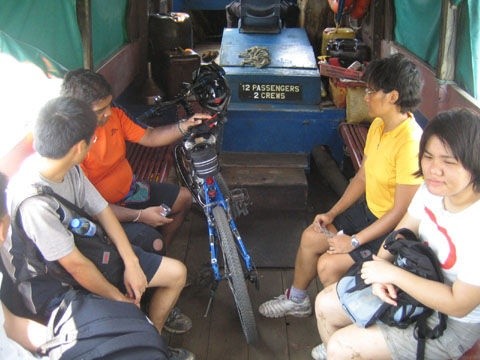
[193,71,233,116]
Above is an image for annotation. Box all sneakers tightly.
[145,302,192,334]
[258,289,312,319]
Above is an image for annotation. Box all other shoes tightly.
[167,346,196,360]
[311,343,327,360]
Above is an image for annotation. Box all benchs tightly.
[338,122,480,360]
[0,120,174,360]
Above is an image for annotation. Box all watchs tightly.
[351,234,360,248]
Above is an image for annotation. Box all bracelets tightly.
[179,119,192,137]
[133,210,141,222]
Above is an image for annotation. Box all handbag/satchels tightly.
[119,175,151,206]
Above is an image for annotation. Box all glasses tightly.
[364,87,382,97]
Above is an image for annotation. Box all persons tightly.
[311,108,480,360]
[226,0,289,28]
[259,55,425,317]
[0,172,172,360]
[0,97,194,360]
[60,69,213,333]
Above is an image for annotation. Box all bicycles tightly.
[132,45,264,345]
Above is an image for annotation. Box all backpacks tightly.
[336,227,449,340]
[10,184,124,291]
